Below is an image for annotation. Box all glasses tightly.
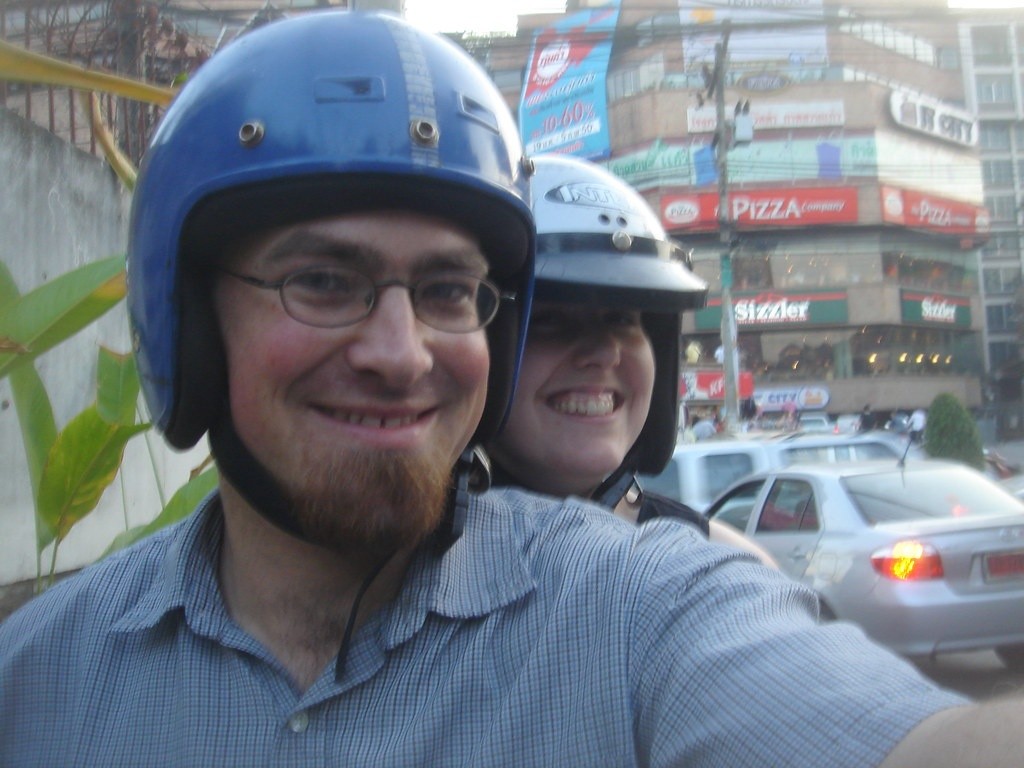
[208,264,514,333]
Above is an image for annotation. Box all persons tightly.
[689,399,926,444]
[461,148,780,575]
[0,9,1024,767]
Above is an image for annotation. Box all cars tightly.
[632,416,923,525]
[700,457,1024,675]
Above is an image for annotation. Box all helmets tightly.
[526,155,706,477]
[125,10,537,451]
